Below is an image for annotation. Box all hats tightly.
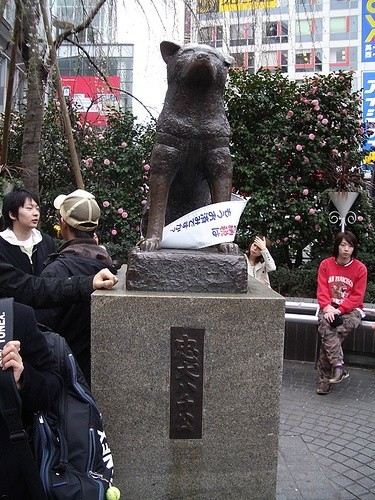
[54,189,100,231]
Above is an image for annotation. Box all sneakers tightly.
[317,377,333,394]
[329,367,349,384]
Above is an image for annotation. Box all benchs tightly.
[285,302,375,369]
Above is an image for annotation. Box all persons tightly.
[316,232,367,396]
[0,189,118,500]
[244,234,276,289]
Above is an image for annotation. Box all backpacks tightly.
[0,298,114,500]
